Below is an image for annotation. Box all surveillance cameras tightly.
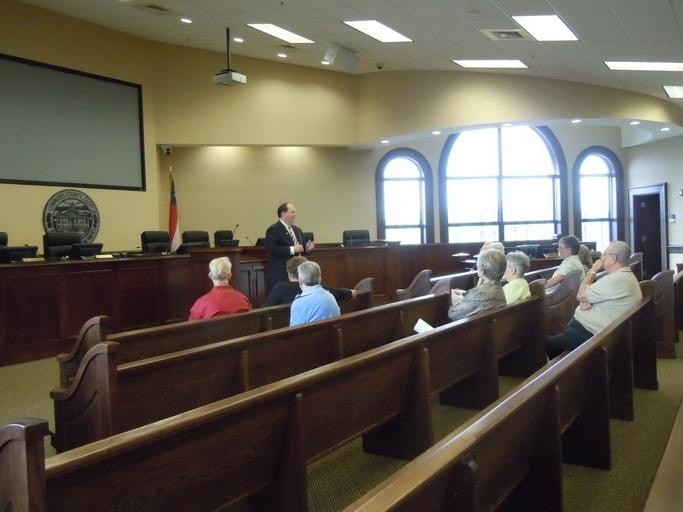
[163,146,172,156]
[377,65,383,70]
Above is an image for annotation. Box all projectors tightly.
[212,71,247,87]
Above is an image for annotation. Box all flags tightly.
[166,173,182,255]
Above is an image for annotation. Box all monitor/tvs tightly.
[182,242,210,254]
[256,238,266,247]
[70,243,103,260]
[0,246,38,263]
[216,240,239,248]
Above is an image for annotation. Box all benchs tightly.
[500,265,560,284]
[650,263,683,360]
[57,277,376,389]
[396,269,479,299]
[50,277,452,454]
[546,252,644,332]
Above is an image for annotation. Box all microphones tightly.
[246,236,255,246]
[232,224,239,236]
[25,244,29,247]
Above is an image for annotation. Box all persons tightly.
[265,202,316,284]
[447,236,644,351]
[290,261,341,326]
[264,256,359,306]
[189,256,253,321]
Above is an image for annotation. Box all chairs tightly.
[0,232,8,247]
[516,244,541,258]
[181,231,210,248]
[255,238,266,246]
[43,232,82,260]
[581,242,596,252]
[214,230,233,247]
[343,230,370,246]
[303,232,314,242]
[141,231,171,254]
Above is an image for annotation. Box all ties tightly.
[288,226,302,256]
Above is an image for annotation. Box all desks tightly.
[529,257,563,270]
